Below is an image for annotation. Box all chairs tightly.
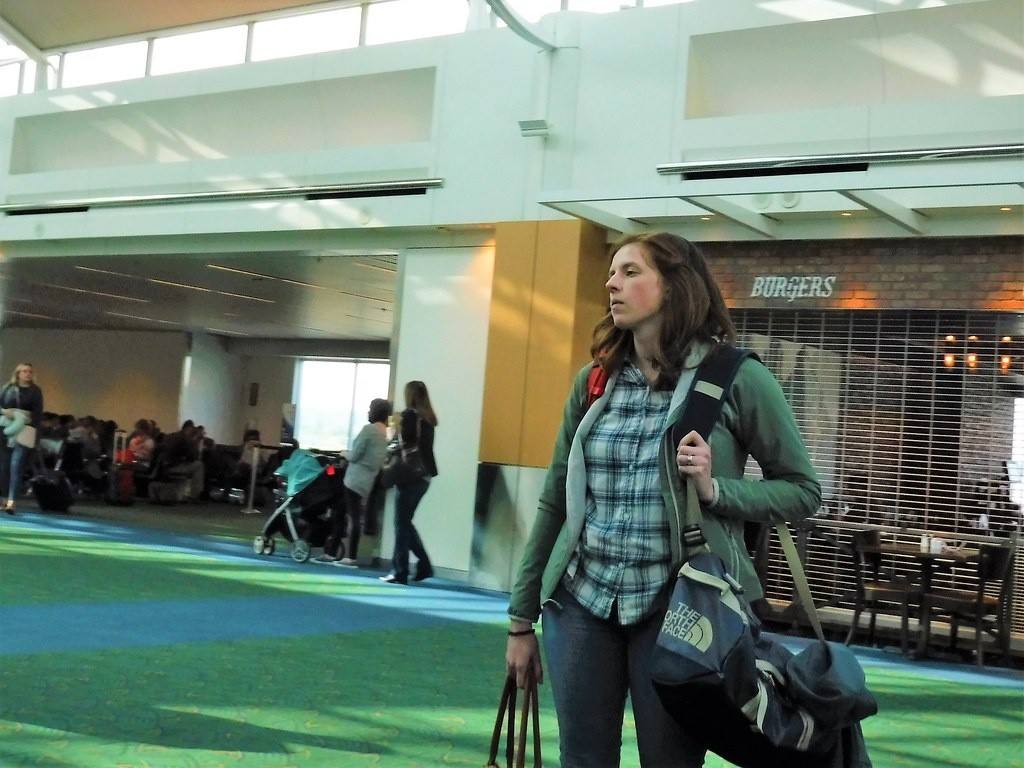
[844,528,919,655]
[918,541,1014,665]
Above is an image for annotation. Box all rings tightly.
[687,456,692,464]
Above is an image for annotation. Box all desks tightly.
[855,544,980,661]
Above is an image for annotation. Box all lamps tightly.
[518,119,553,138]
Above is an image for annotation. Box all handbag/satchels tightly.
[381,446,428,489]
[651,556,848,768]
[17,426,36,449]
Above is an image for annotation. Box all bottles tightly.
[921,534,929,547]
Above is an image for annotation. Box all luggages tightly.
[28,448,78,514]
[108,428,142,505]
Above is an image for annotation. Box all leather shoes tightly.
[379,574,404,584]
[408,570,435,581]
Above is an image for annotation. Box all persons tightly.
[378,380,439,583]
[505,231,823,768]
[830,465,880,567]
[41,411,156,472]
[239,430,261,467]
[0,363,44,515]
[160,419,205,499]
[309,398,392,569]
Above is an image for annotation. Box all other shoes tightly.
[334,557,359,570]
[308,554,338,566]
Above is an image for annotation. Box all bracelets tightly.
[508,628,535,637]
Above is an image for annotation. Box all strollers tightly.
[253,449,350,563]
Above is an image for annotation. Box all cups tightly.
[930,540,942,549]
